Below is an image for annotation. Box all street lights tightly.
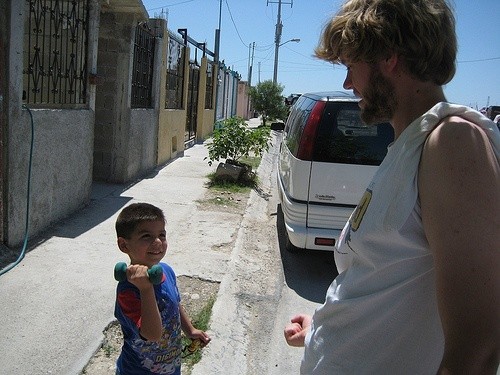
[280,38,301,46]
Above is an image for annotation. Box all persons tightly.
[283,0,500,375]
[114,203,212,375]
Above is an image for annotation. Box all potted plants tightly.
[204,116,273,179]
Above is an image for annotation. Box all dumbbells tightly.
[114,262,163,284]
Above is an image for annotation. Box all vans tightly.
[277,90,395,254]
